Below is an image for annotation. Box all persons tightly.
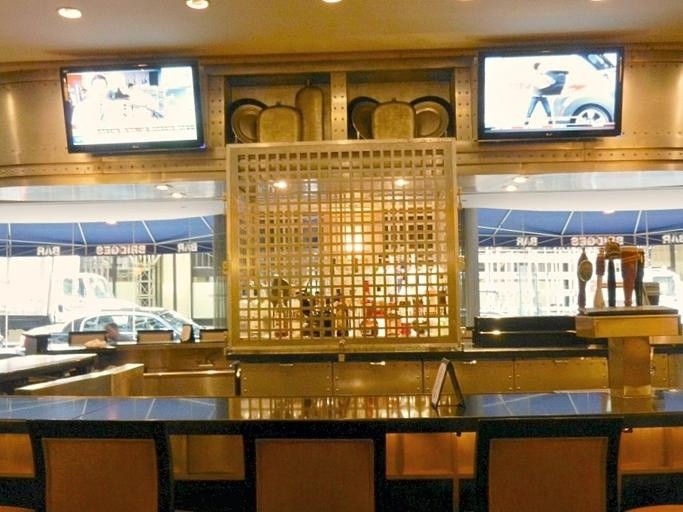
[100,321,132,344]
[519,63,557,128]
[69,76,129,137]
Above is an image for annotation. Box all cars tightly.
[28,307,210,345]
[549,70,616,129]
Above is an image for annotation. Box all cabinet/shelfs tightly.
[0,343,682,511]
[205,56,474,157]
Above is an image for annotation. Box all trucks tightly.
[0,255,115,324]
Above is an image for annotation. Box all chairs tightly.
[136,330,173,343]
[69,332,108,346]
[199,329,227,342]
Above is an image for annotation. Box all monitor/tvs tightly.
[58,60,206,154]
[477,44,624,143]
